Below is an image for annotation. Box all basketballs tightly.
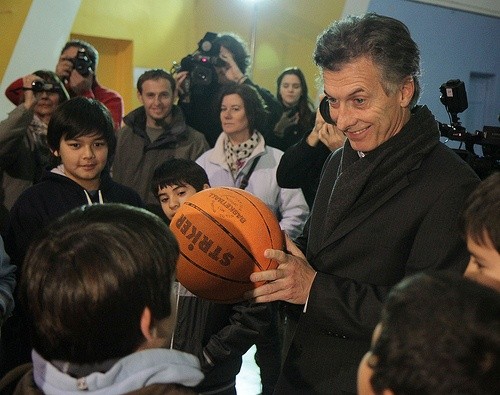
[170,185,286,305]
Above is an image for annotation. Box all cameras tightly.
[180,32,226,88]
[31,81,63,92]
[64,49,93,77]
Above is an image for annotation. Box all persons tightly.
[243,12,480,395]
[0,32,500,395]
